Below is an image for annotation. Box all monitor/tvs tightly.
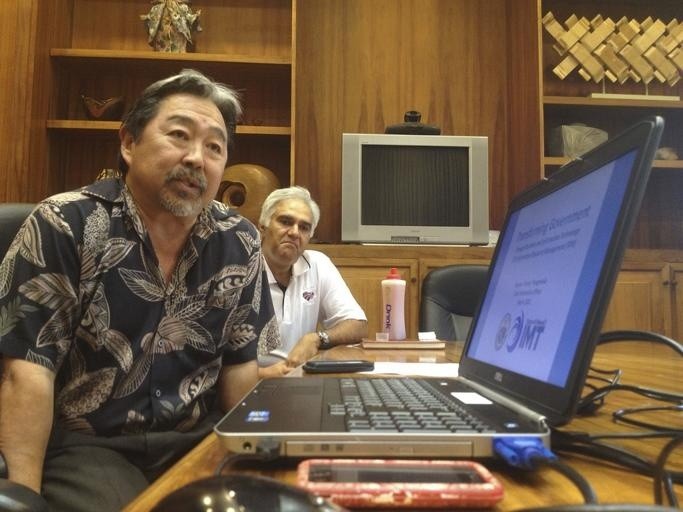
[342,133,489,247]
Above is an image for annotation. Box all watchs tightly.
[318,330,330,349]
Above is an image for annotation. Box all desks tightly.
[122,334,682,512]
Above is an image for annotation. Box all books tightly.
[361,339,446,349]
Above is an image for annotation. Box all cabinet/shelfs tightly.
[590,250,680,355]
[44,0,296,203]
[512,1,683,181]
[331,254,418,342]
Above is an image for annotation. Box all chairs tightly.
[419,263,494,344]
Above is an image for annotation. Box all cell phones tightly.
[296,458,504,509]
[302,359,374,373]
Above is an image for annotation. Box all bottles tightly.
[380,267,407,341]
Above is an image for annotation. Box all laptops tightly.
[213,116,665,459]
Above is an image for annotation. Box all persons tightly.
[0,69,282,511]
[257,186,368,379]
